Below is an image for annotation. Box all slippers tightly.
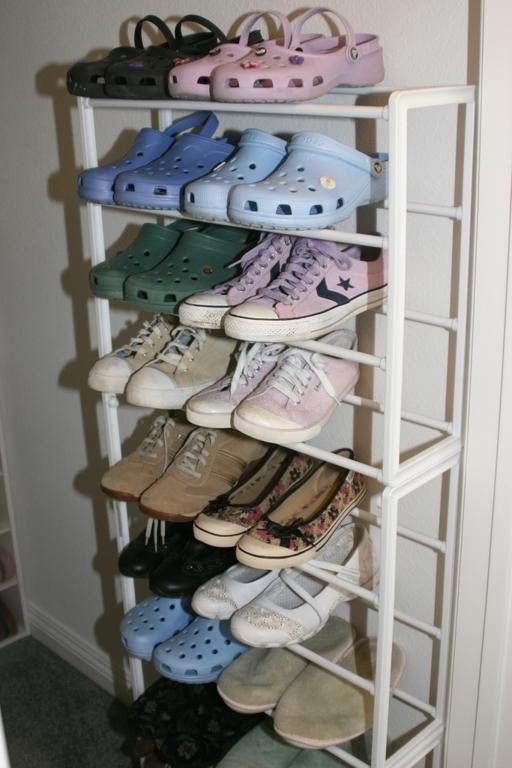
[125,673,371,766]
[271,635,407,751]
[215,615,357,714]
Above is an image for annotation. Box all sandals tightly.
[64,5,391,240]
[118,591,249,686]
[84,213,251,316]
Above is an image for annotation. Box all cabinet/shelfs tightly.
[0,431,32,652]
[55,72,480,767]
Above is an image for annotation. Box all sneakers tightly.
[178,234,390,345]
[88,313,381,651]
